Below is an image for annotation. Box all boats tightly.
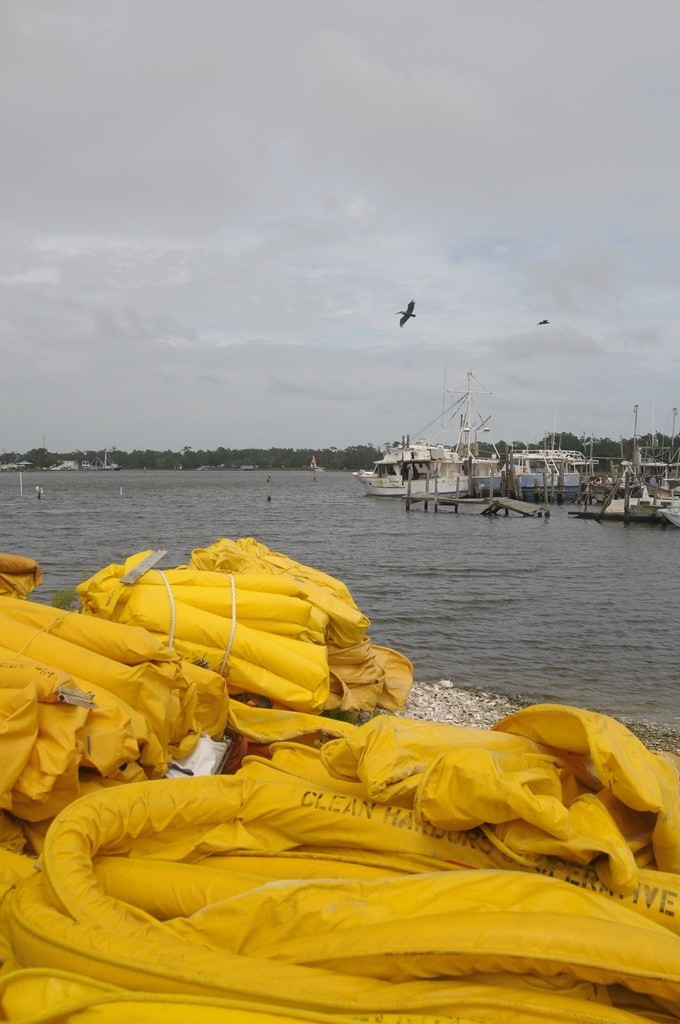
[352,359,498,498]
[593,383,680,511]
[659,506,680,529]
[466,407,599,499]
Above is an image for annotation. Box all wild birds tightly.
[537,320,550,326]
[394,299,417,327]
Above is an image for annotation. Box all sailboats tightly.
[102,448,122,470]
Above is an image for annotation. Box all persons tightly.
[649,475,657,486]
